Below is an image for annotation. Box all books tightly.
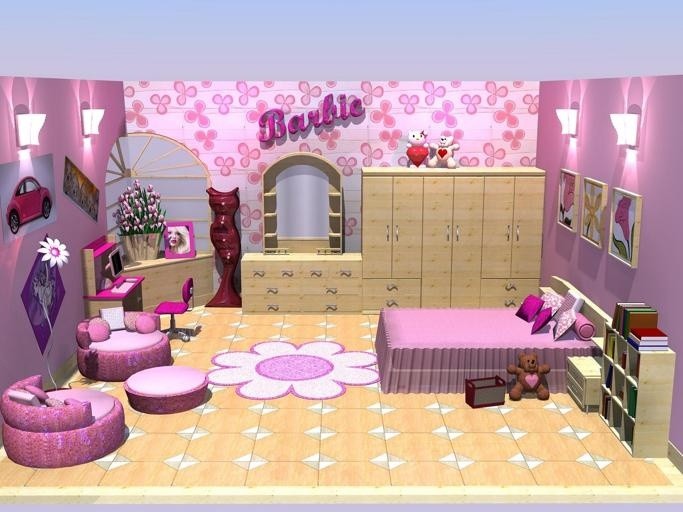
[600,300,669,438]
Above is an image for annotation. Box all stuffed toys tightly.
[507,351,551,401]
[406,130,430,170]
[429,135,460,168]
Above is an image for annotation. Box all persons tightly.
[166,225,191,253]
